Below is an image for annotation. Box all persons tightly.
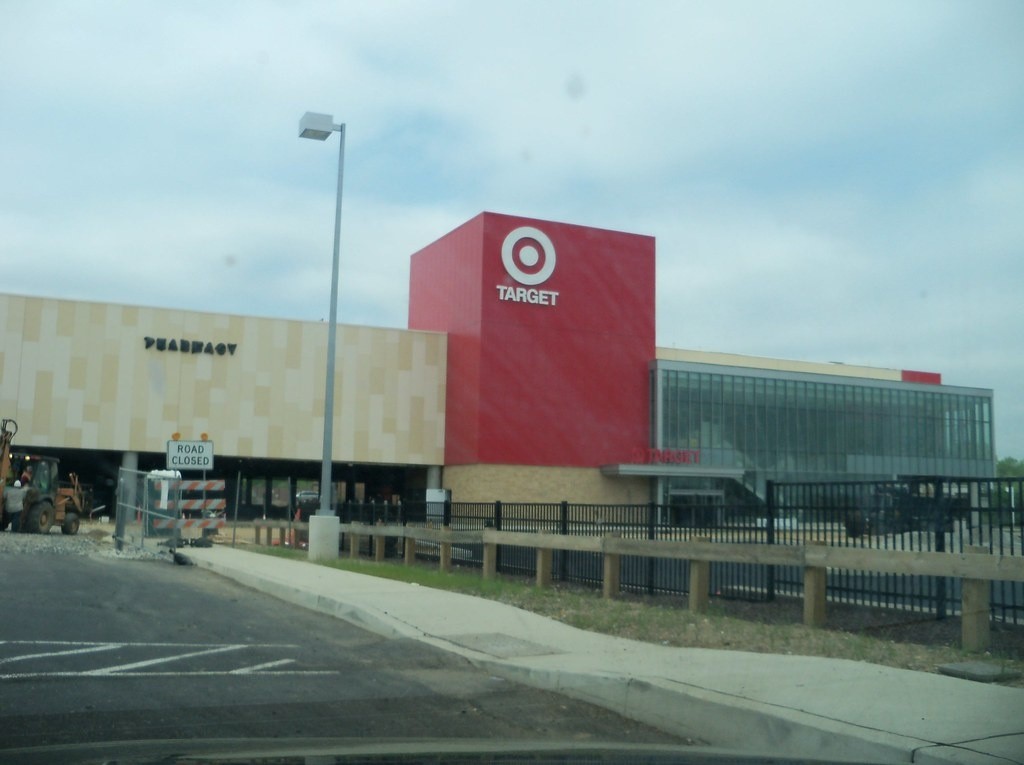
[21,466,32,486]
[0,480,29,533]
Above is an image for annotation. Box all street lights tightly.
[295,111,346,515]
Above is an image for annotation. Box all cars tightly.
[295,491,318,504]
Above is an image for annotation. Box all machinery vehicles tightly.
[0,416,87,535]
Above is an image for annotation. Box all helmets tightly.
[14,480,21,487]
